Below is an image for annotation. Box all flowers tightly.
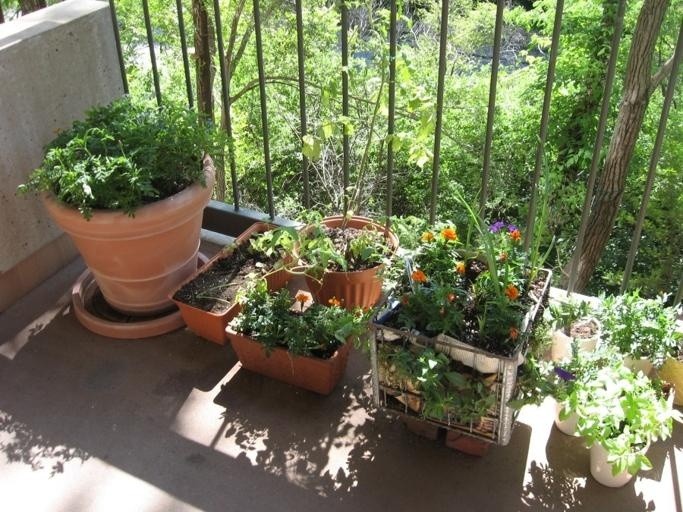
[386,207,536,352]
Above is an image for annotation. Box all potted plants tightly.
[14,102,236,340]
[543,288,683,487]
[168,103,397,397]
[373,339,499,457]
